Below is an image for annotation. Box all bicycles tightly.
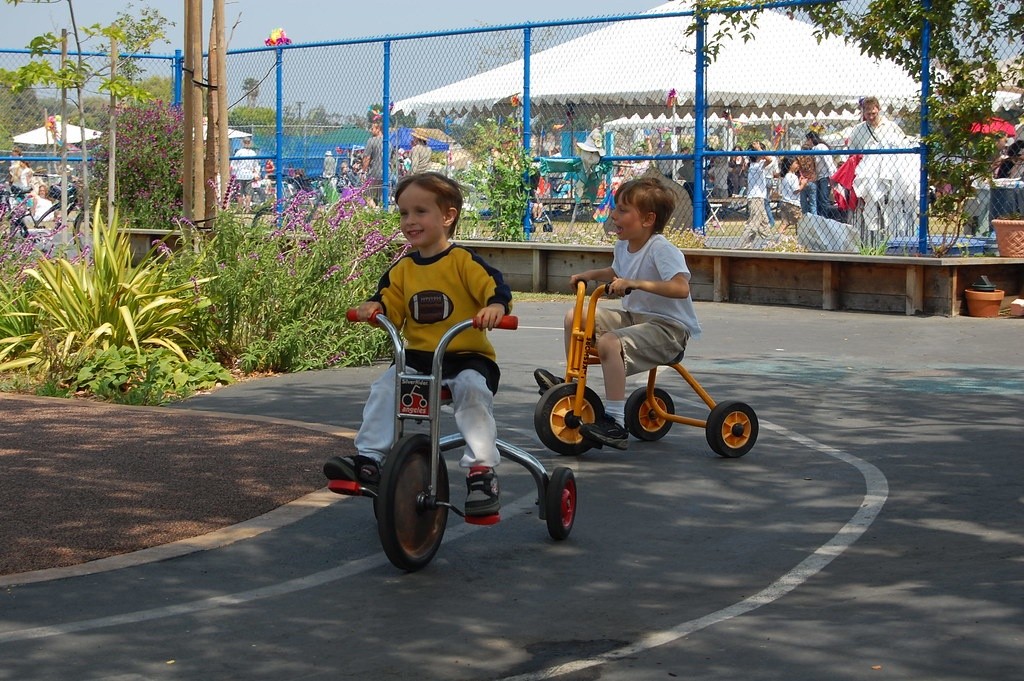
[0,178,99,254]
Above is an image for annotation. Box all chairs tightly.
[728,187,746,208]
[705,188,713,198]
[684,182,724,233]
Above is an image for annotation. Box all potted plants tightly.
[992,212,1024,257]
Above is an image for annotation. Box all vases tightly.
[965,289,1004,317]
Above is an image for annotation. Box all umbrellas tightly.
[966,116,1016,138]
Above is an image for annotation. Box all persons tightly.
[264,159,274,178]
[323,173,513,517]
[230,136,261,214]
[620,95,1024,248]
[534,177,701,448]
[8,147,54,221]
[323,119,433,198]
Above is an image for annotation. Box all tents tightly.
[388,2,1024,189]
[192,119,253,149]
[12,118,102,153]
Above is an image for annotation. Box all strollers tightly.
[521,166,553,232]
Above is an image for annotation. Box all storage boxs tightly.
[880,234,999,257]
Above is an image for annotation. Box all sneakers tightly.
[579,415,630,451]
[534,369,565,391]
[464,467,501,515]
[323,455,380,494]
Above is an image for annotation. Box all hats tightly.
[241,136,253,148]
[410,127,430,141]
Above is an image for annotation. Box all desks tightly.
[707,197,783,218]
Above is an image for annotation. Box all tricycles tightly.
[534,277,760,458]
[328,308,577,571]
